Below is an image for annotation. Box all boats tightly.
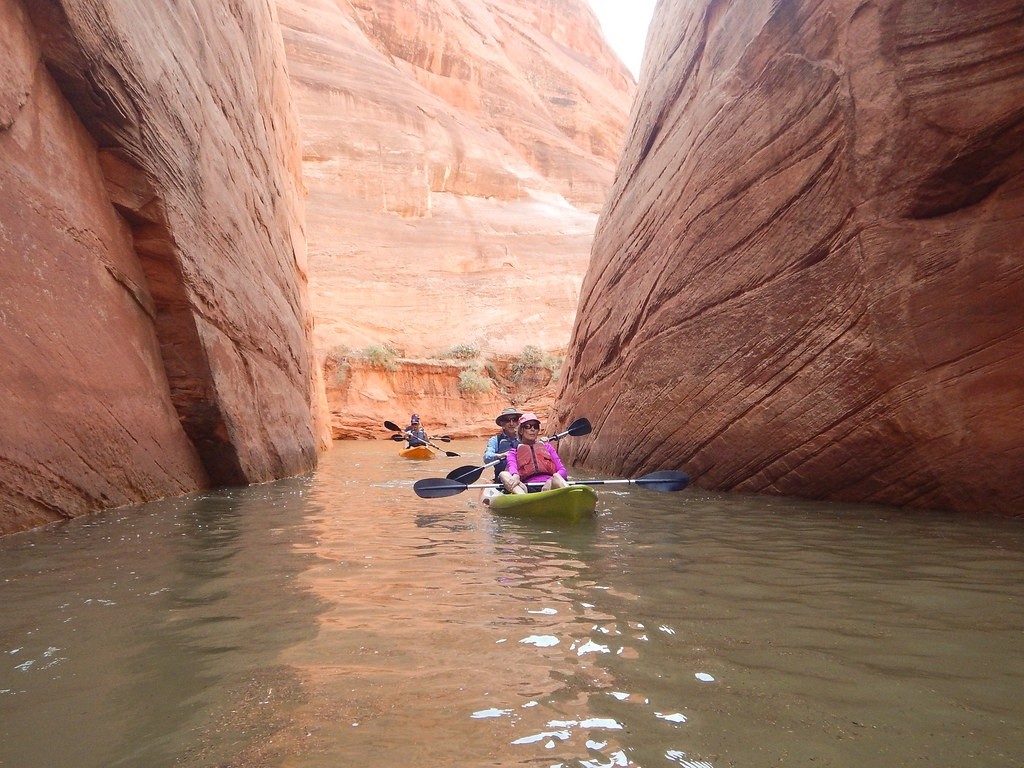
[400,446,436,460]
[480,484,600,525]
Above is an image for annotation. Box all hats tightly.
[519,412,541,426]
[411,418,419,424]
[412,414,420,419]
[495,408,523,426]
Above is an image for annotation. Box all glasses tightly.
[520,423,539,430]
[500,416,518,423]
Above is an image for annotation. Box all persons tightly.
[398,414,429,449]
[498,414,567,494]
[483,408,549,491]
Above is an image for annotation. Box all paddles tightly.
[390,434,452,443]
[383,420,460,457]
[413,470,689,500]
[446,417,593,485]
